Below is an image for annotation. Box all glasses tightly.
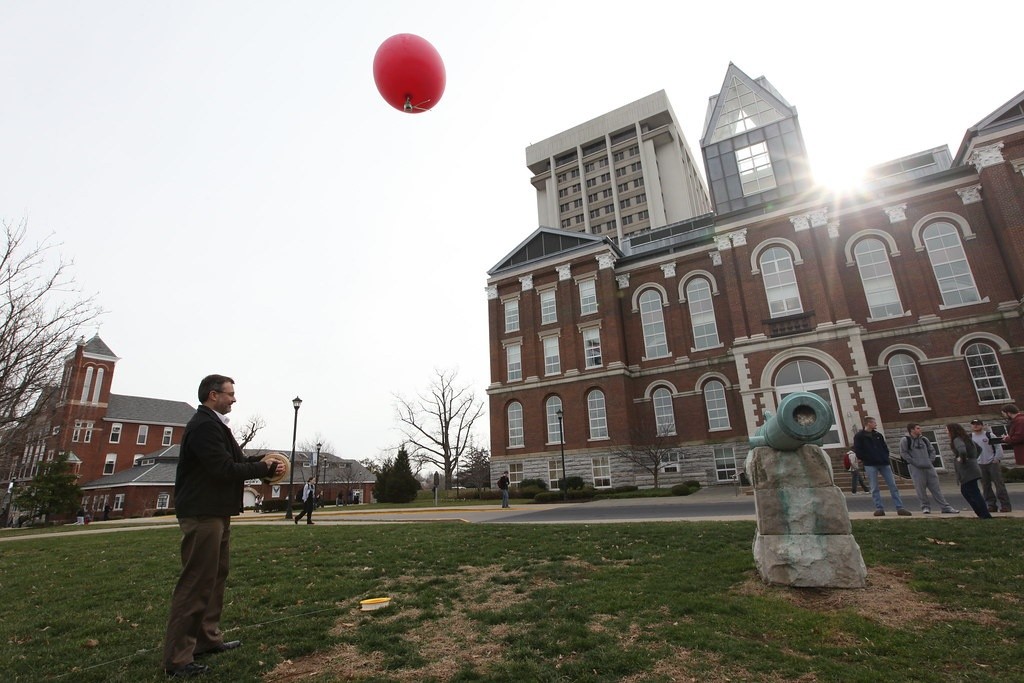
[217,390,234,397]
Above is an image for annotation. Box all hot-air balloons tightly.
[372,33,446,114]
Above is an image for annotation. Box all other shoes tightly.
[163,663,214,676]
[922,507,930,514]
[307,522,314,524]
[295,517,297,524]
[941,506,960,513]
[874,510,885,516]
[195,640,242,650]
[897,509,912,516]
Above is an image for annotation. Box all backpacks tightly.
[844,451,853,470]
[296,483,309,502]
[497,480,503,488]
[899,435,928,465]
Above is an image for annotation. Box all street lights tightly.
[454,446,462,498]
[4,474,17,527]
[556,409,569,503]
[323,456,327,507]
[313,442,322,509]
[286,395,303,520]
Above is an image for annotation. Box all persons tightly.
[76,502,111,521]
[899,423,960,514]
[846,446,870,495]
[254,495,265,513]
[966,419,1012,513]
[945,423,993,520]
[295,477,344,525]
[852,416,912,516]
[163,374,286,677]
[1001,403,1024,467]
[501,471,510,508]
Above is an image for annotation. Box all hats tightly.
[971,418,984,425]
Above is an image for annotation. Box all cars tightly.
[451,486,466,490]
